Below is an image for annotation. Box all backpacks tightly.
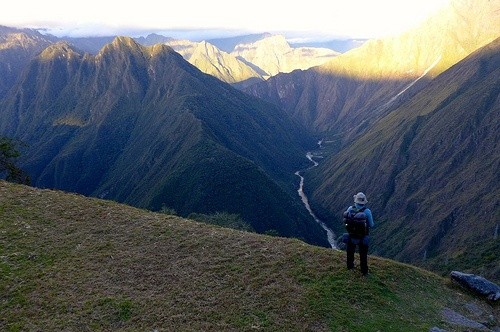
[343,208,369,239]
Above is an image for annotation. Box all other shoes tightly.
[361,271,369,276]
[347,263,355,271]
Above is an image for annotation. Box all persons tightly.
[343,193,374,275]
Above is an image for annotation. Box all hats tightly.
[354,191,368,205]
